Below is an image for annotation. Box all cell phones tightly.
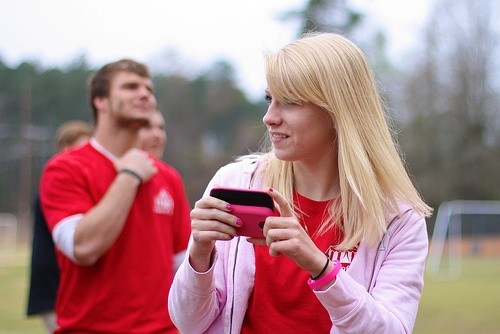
[210,188,280,239]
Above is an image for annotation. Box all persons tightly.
[27,123,95,334]
[39,59,193,334]
[137,108,167,159]
[168,33,435,334]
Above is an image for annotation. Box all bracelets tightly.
[119,168,143,184]
[307,260,342,290]
[310,253,330,281]
